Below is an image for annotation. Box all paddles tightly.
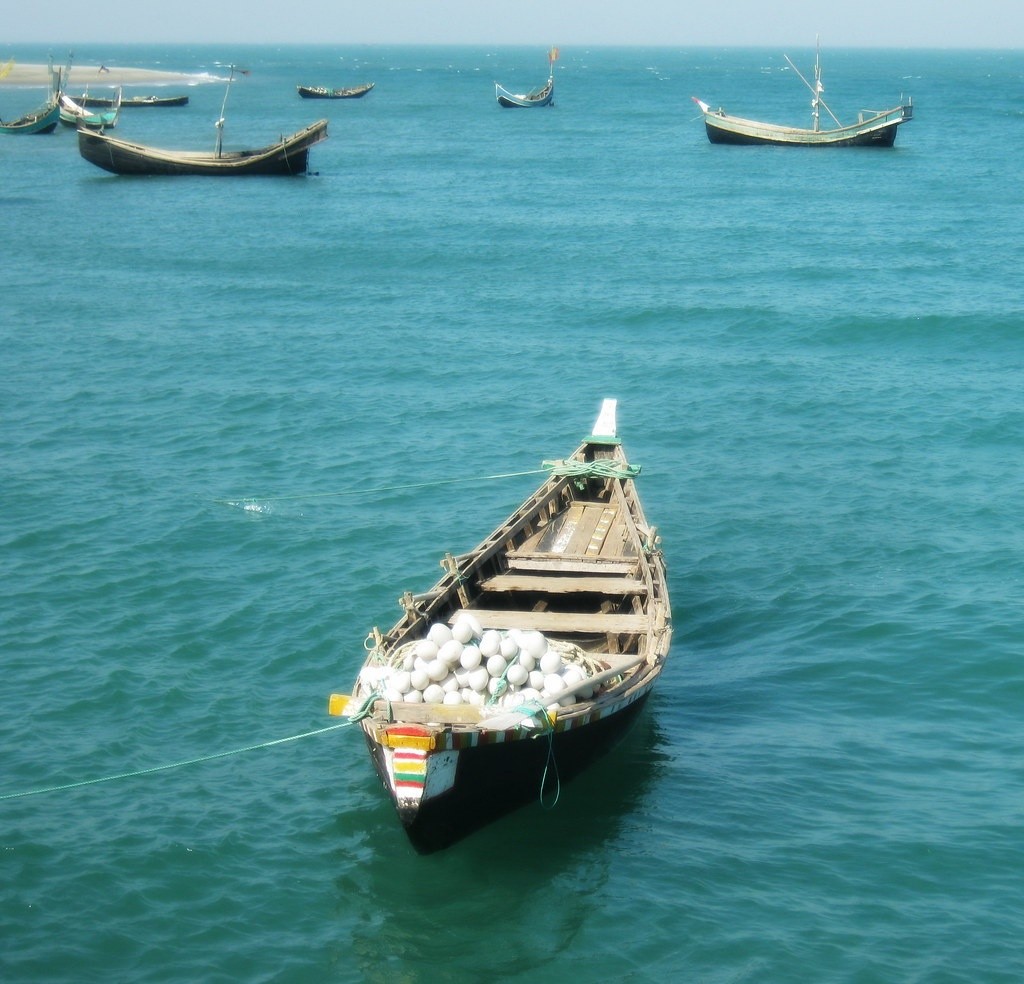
[473,654,646,732]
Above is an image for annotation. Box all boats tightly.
[296,83,376,98]
[0,49,122,134]
[494,45,554,108]
[76,62,329,175]
[328,400,672,855]
[692,33,914,148]
[68,95,188,107]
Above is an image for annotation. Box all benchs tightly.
[447,609,650,634]
[480,575,648,595]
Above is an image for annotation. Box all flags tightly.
[692,96,711,113]
[549,48,558,66]
